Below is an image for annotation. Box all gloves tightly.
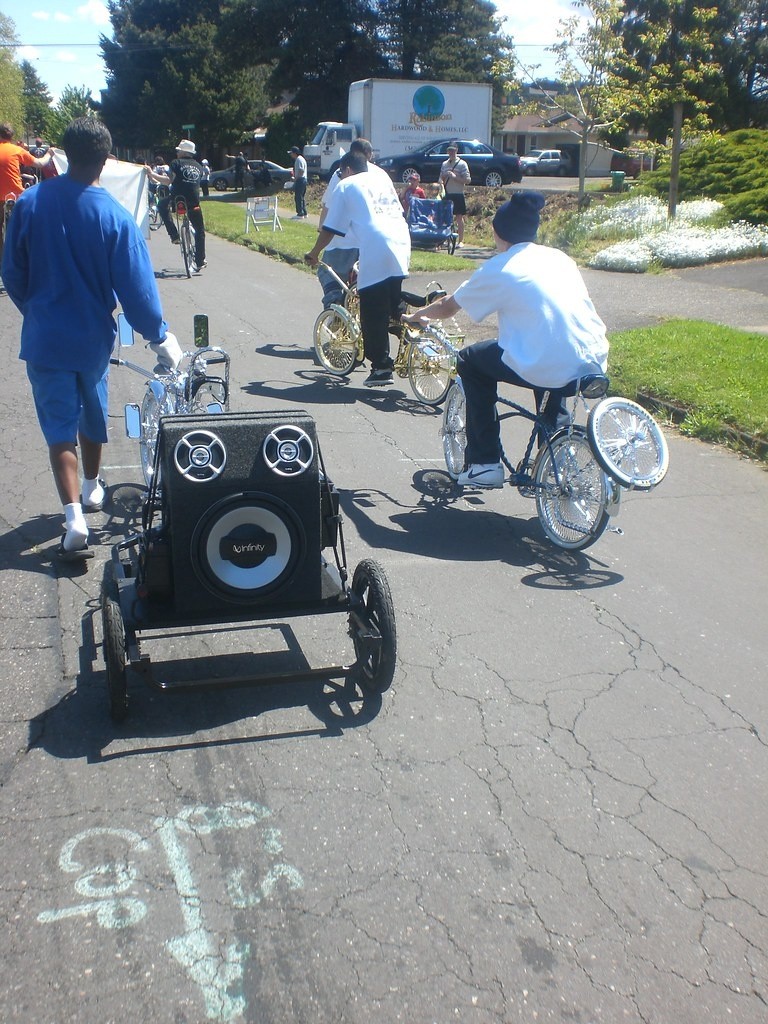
[150,332,183,375]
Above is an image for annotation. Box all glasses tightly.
[336,165,354,178]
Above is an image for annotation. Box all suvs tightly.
[519,149,572,177]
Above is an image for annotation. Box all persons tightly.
[303,151,411,387]
[136,139,208,271]
[3,120,183,562]
[200,158,210,201]
[400,173,426,216]
[426,180,445,201]
[225,152,250,191]
[317,139,374,334]
[438,142,471,248]
[400,192,611,488]
[0,122,60,293]
[287,146,308,219]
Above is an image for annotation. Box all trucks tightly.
[302,78,493,181]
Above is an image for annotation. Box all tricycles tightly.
[102,312,397,724]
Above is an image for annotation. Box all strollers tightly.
[407,197,459,255]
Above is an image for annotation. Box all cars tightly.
[374,137,522,187]
[207,160,294,190]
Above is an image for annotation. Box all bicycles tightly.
[149,184,206,279]
[305,255,467,407]
[406,318,669,553]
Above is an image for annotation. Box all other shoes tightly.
[171,235,179,243]
[292,215,304,219]
[304,215,308,218]
[196,260,207,266]
[444,242,451,247]
[456,242,464,247]
[326,315,340,333]
[0,287,8,297]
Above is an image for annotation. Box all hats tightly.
[447,142,458,150]
[287,146,300,155]
[175,140,196,154]
[408,173,420,182]
[492,191,545,243]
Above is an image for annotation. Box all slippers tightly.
[82,479,108,512]
[61,532,95,562]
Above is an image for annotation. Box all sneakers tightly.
[363,368,394,385]
[456,464,504,488]
[547,475,563,483]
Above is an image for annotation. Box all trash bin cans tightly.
[609,170,626,192]
